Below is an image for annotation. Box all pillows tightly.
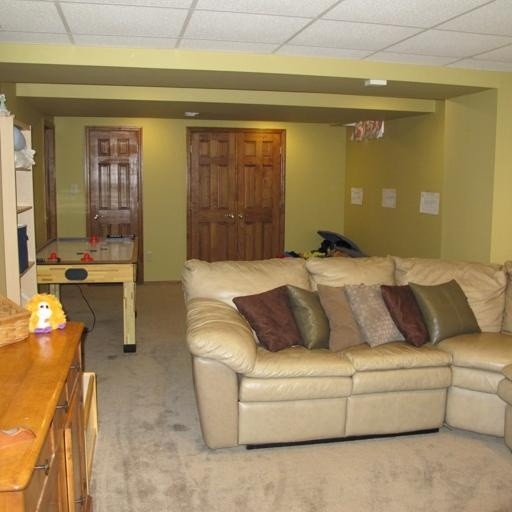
[232,279,482,353]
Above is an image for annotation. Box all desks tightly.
[37,238,138,353]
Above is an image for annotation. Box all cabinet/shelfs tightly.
[0,319,94,512]
[0,114,39,312]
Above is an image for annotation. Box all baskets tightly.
[0,293,35,349]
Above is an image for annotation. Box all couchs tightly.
[182,255,511,451]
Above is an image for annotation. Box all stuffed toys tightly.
[25,328,68,365]
[23,292,68,334]
